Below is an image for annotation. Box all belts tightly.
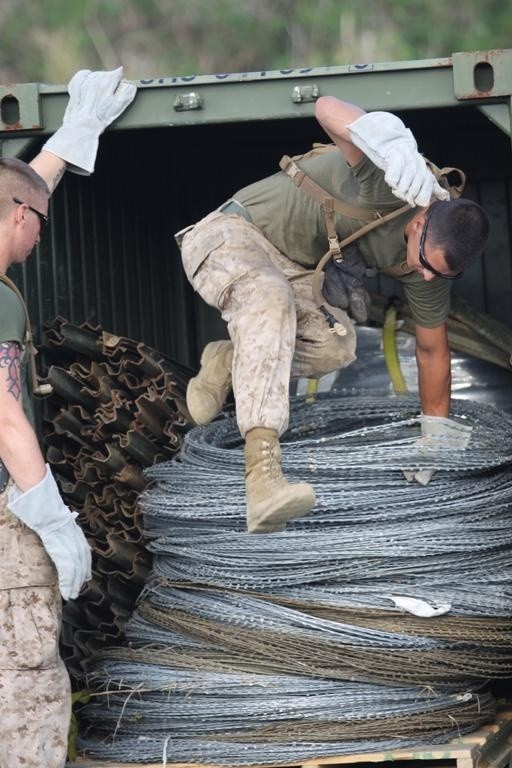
[221,201,252,221]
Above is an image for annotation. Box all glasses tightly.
[419,212,463,279]
[13,198,49,231]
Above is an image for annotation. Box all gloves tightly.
[7,462,93,601]
[42,67,137,176]
[401,413,472,485]
[347,112,451,208]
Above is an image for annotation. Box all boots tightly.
[186,341,235,428]
[243,429,315,535]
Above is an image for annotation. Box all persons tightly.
[174,96,490,535]
[0,63,139,767]
